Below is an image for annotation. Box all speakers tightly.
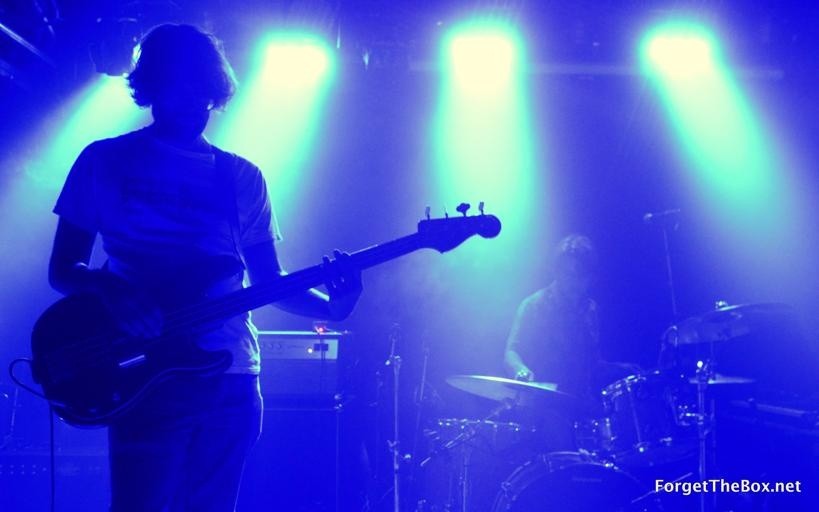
[241,397,352,512]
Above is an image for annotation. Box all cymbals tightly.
[667,300,793,345]
[687,371,758,385]
[444,374,585,415]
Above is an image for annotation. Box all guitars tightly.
[30,201,501,431]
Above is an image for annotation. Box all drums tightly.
[601,368,698,479]
[574,415,612,463]
[491,450,664,512]
[417,418,524,511]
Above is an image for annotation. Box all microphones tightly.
[643,207,682,224]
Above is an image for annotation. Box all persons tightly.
[502,234,643,407]
[45,20,364,512]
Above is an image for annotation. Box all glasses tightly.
[153,96,215,111]
